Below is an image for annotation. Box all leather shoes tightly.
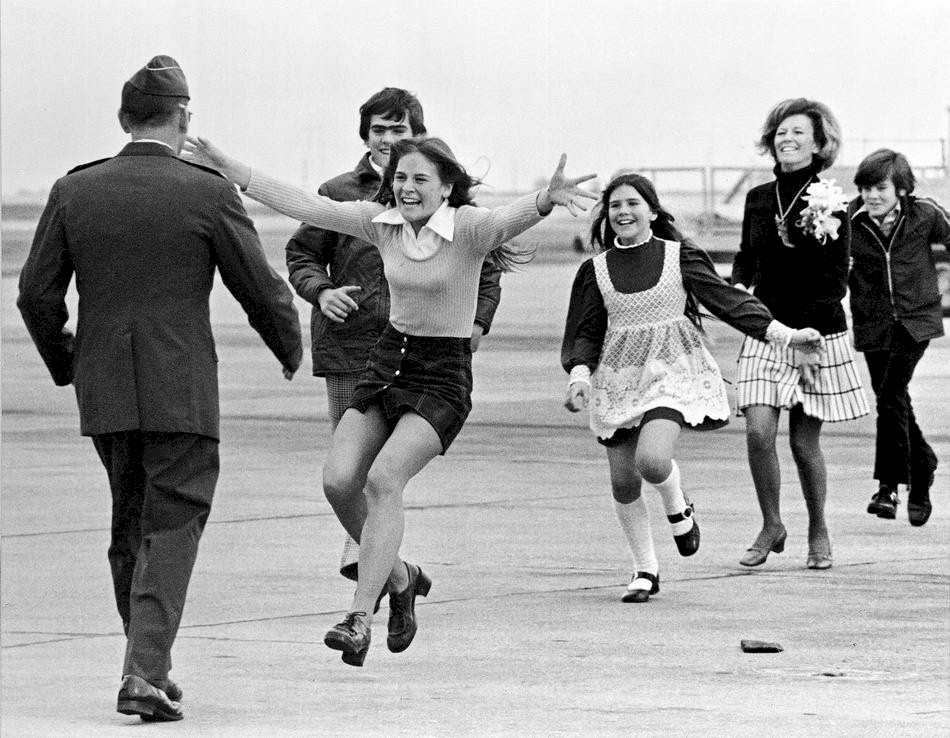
[116,675,185,720]
[165,680,183,701]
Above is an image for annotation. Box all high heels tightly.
[386,561,431,653]
[325,611,373,667]
[807,538,832,568]
[740,524,787,566]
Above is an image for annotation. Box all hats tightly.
[129,56,190,100]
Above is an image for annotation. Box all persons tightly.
[845,147,950,526]
[561,173,829,602]
[284,88,503,615]
[179,136,598,666]
[731,97,870,569]
[15,55,304,723]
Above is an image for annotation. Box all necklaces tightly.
[774,175,813,247]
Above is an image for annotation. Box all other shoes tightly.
[666,497,701,556]
[621,571,660,603]
[907,469,934,526]
[867,488,901,519]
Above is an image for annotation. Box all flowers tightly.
[800,174,850,242]
